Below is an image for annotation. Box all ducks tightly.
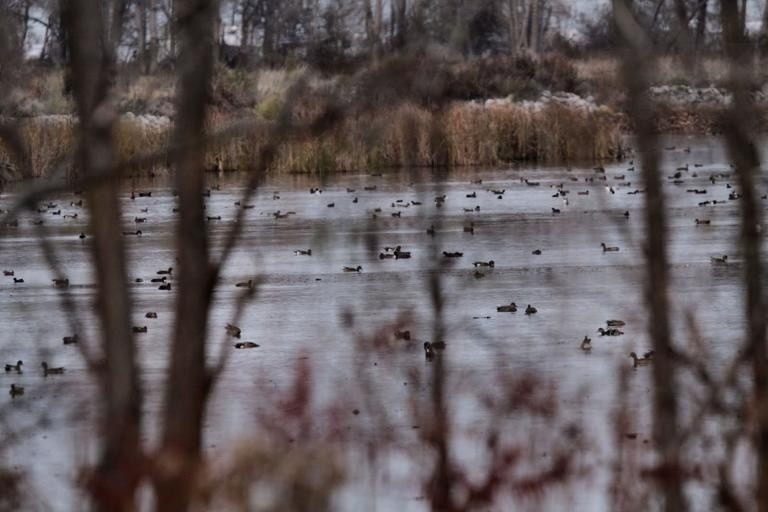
[4,359,23,375]
[666,144,677,149]
[627,352,653,367]
[684,146,691,153]
[9,383,24,396]
[0,163,741,352]
[41,359,64,376]
